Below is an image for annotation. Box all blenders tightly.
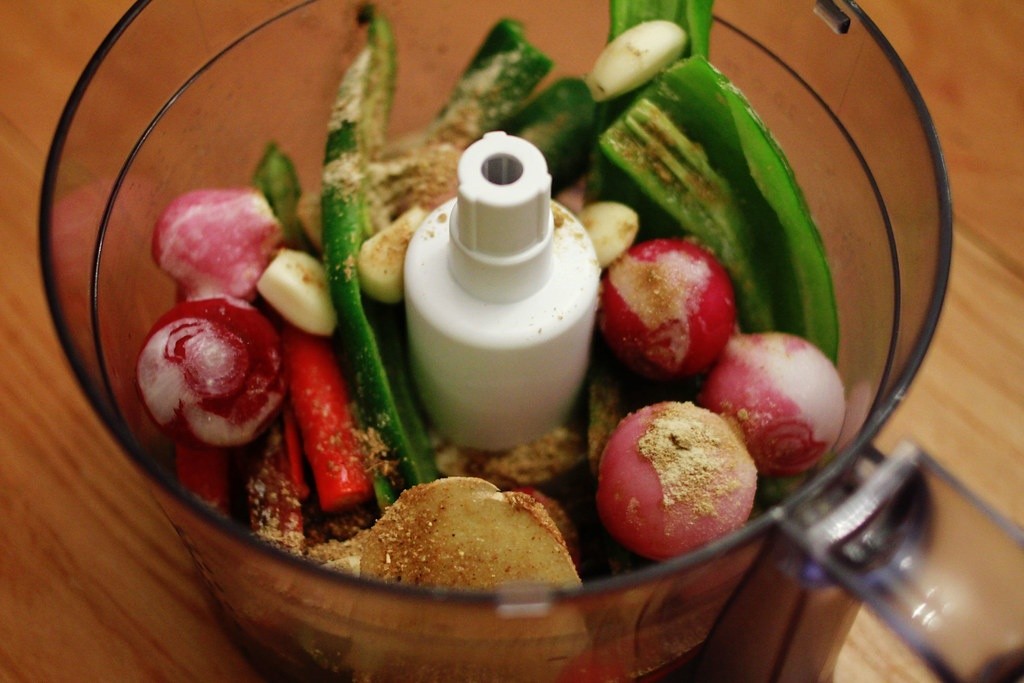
[36,0,1024,683]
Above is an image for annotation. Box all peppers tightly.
[171,0,842,557]
